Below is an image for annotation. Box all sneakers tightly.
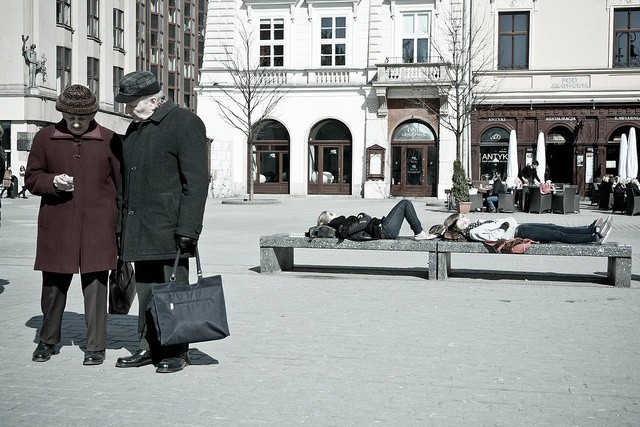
[592,216,603,227]
[595,226,612,245]
[595,215,612,238]
[415,230,438,240]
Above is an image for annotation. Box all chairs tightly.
[527,186,552,214]
[468,194,482,211]
[625,188,640,216]
[498,187,518,213]
[473,181,489,206]
[598,184,614,210]
[552,186,577,215]
[591,183,601,205]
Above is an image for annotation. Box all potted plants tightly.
[451,160,472,214]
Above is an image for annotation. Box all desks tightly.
[476,187,490,194]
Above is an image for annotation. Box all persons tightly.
[0,165,14,198]
[17,165,28,198]
[443,241,445,243]
[23,83,123,366]
[444,214,614,245]
[486,174,507,213]
[520,160,543,212]
[317,199,438,241]
[113,70,211,375]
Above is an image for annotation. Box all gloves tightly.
[175,236,197,255]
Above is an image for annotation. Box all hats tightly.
[115,71,161,102]
[56,85,99,115]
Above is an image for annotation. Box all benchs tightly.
[437,238,632,289]
[259,230,442,281]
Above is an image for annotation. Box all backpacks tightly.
[305,226,336,243]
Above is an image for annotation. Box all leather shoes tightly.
[156,350,189,372]
[115,349,154,367]
[32,341,56,362]
[83,351,106,365]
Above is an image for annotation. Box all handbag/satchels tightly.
[110,234,136,315]
[148,244,231,346]
